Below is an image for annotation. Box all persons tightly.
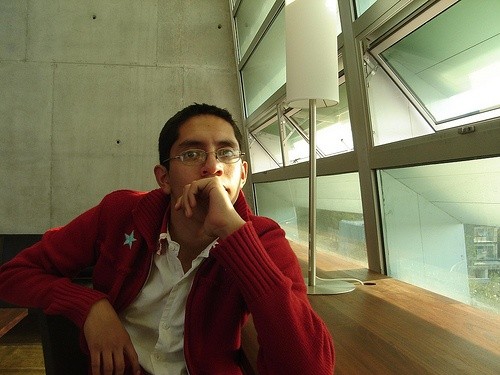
[0,102,336,375]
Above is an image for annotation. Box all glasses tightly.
[162,146,246,166]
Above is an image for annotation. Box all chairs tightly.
[39,312,88,375]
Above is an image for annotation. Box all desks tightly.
[241,239,500,375]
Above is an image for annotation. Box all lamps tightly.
[285,0,356,294]
